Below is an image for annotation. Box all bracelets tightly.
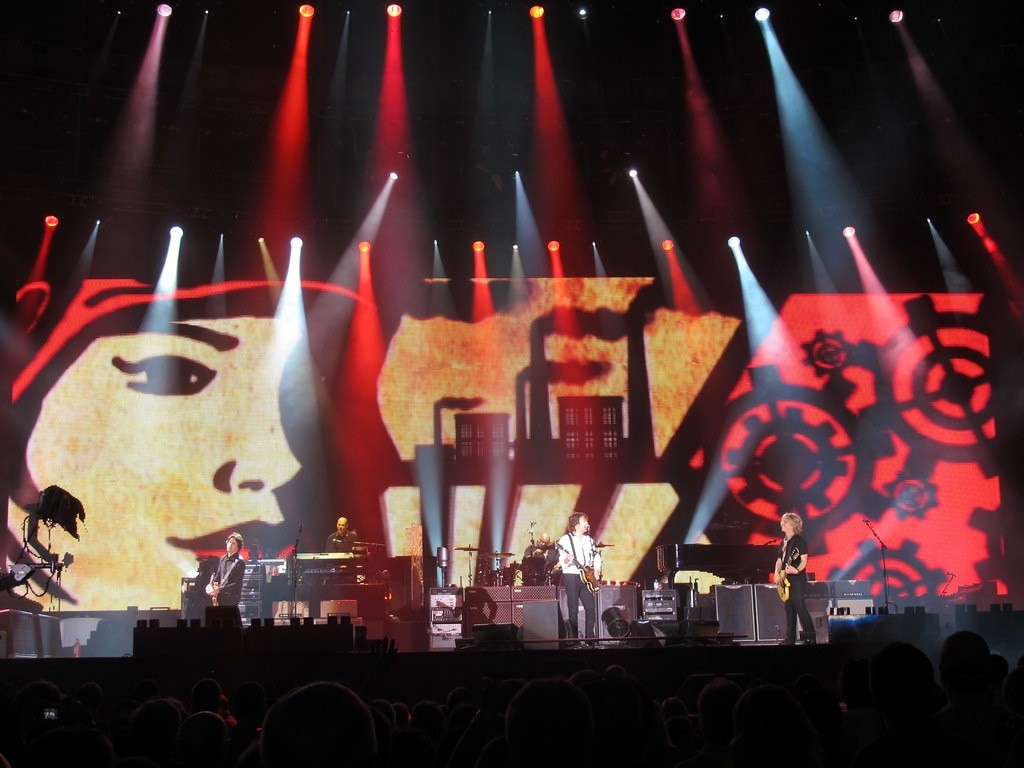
[796,569,801,575]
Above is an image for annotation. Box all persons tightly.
[521,533,559,587]
[557,511,602,646]
[775,513,817,647]
[0,642,1024,768]
[325,516,358,625]
[205,532,246,632]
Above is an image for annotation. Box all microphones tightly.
[584,524,590,529]
[862,519,870,522]
[332,539,341,542]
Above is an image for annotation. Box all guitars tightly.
[210,573,218,606]
[529,521,546,557]
[554,540,602,593]
[777,547,800,602]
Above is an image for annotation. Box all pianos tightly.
[655,543,780,590]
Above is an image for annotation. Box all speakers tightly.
[709,584,756,641]
[473,623,518,639]
[796,598,834,643]
[512,601,523,628]
[557,586,599,645]
[465,586,510,601]
[753,584,798,641]
[834,597,874,614]
[679,620,720,642]
[625,619,680,645]
[34,614,64,658]
[465,602,512,638]
[597,586,637,645]
[511,586,556,602]
[0,608,39,658]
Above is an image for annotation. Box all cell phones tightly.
[43,709,58,719]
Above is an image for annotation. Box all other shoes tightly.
[779,638,796,646]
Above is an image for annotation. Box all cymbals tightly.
[454,545,486,551]
[481,551,515,558]
